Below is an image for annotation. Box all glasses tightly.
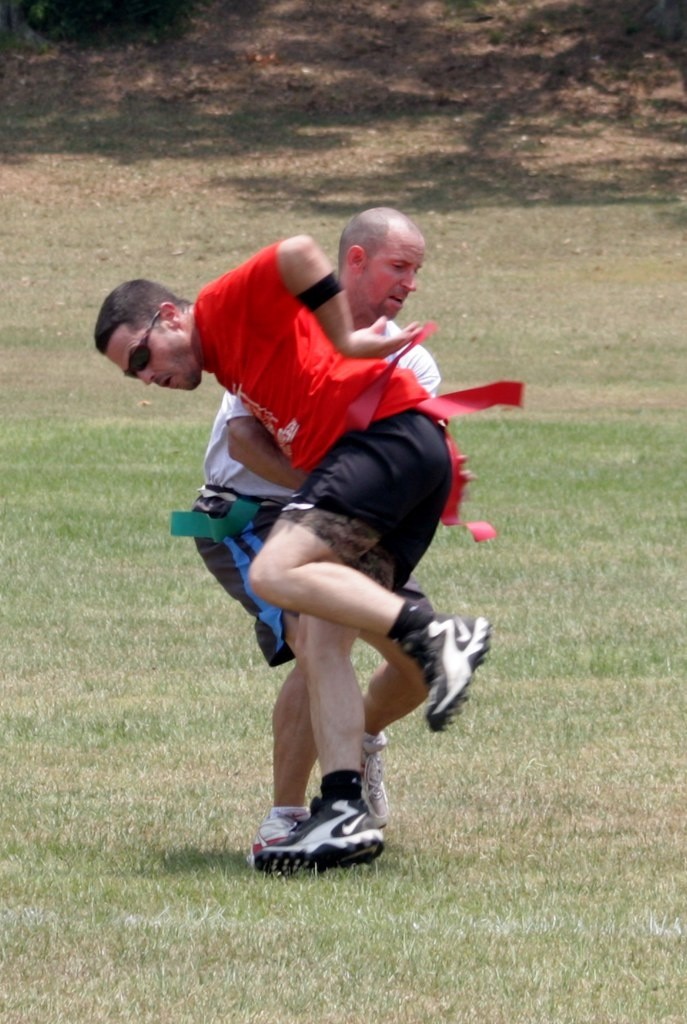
[123,310,162,380]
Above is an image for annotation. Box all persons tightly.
[94,235,491,874]
[170,207,441,866]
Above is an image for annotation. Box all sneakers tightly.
[254,797,383,873]
[403,612,491,732]
[358,729,390,830]
[245,807,312,870]
